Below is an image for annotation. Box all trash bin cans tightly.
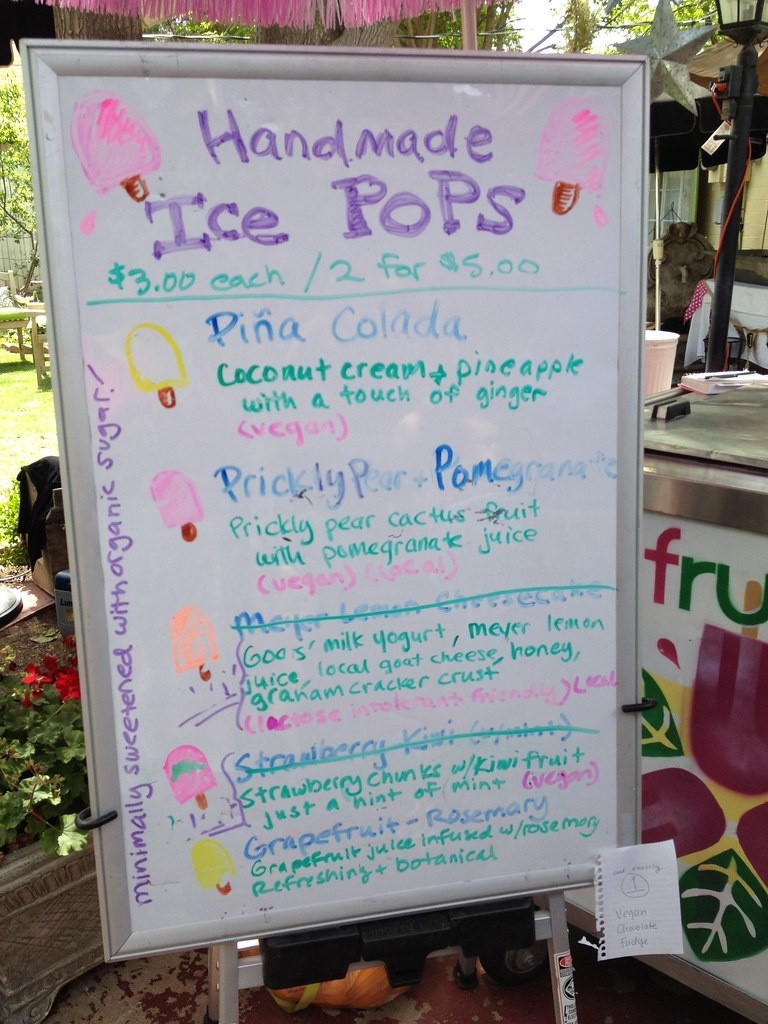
[645,330,680,396]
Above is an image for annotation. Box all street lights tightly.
[705,0,768,373]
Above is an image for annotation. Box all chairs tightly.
[0,270,34,364]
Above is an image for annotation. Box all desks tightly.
[21,308,51,385]
[683,278,768,370]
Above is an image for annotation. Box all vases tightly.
[0,830,105,1024]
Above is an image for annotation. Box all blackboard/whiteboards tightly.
[21,40,651,965]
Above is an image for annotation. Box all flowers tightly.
[0,633,91,858]
